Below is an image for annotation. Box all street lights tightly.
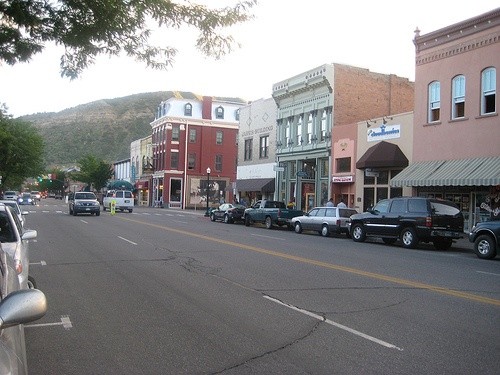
[206,167,211,217]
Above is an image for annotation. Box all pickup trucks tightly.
[243,199,303,229]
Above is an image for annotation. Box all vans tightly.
[103,190,135,212]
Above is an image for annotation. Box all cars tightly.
[0,200,31,227]
[0,243,49,374]
[17,191,62,205]
[468,221,500,259]
[0,203,39,289]
[209,203,246,224]
[1,191,20,202]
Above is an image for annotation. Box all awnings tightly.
[134,181,148,190]
[224,178,275,193]
[390,155,500,186]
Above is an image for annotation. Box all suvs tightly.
[347,195,467,250]
[291,207,360,237]
[69,191,100,216]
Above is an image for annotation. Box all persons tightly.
[321,198,347,208]
[160,196,162,208]
[233,197,255,208]
[287,199,295,209]
